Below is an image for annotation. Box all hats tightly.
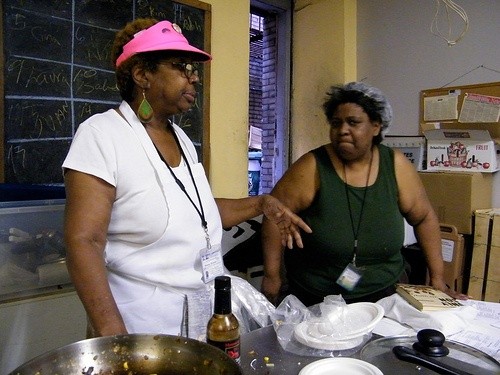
[114,20,213,65]
[344,81,393,140]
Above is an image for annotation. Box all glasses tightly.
[150,62,204,79]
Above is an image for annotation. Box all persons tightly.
[260,80,473,308]
[62,16,312,343]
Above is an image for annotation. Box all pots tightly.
[7,333,244,375]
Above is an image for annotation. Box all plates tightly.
[293,302,384,350]
[297,357,383,375]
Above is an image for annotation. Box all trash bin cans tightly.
[244,149,263,195]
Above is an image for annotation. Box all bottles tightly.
[207,276,241,368]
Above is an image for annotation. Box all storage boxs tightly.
[384,123,500,304]
[0,204,73,302]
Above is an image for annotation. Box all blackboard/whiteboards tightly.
[0,1,211,203]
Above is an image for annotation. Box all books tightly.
[395,283,465,313]
